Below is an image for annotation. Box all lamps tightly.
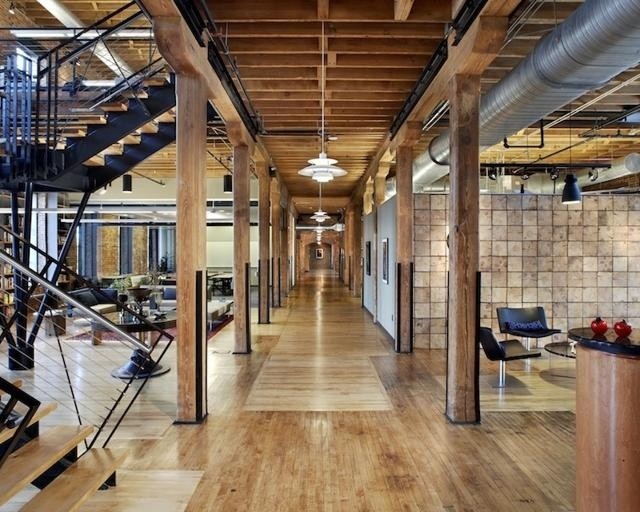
[297,20,349,246]
[480,102,614,205]
[8,1,16,15]
[122,169,132,193]
[222,174,234,193]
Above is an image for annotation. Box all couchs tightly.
[66,285,207,311]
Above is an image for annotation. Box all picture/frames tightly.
[316,249,323,259]
[366,238,389,284]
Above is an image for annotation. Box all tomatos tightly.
[591,317,607,335]
[614,319,632,337]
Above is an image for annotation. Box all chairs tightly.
[478,306,561,388]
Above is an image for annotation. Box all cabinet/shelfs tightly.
[0,225,24,317]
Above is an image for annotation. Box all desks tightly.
[100,272,234,296]
[568,326,640,512]
[89,304,196,344]
[544,342,577,379]
[33,310,66,336]
